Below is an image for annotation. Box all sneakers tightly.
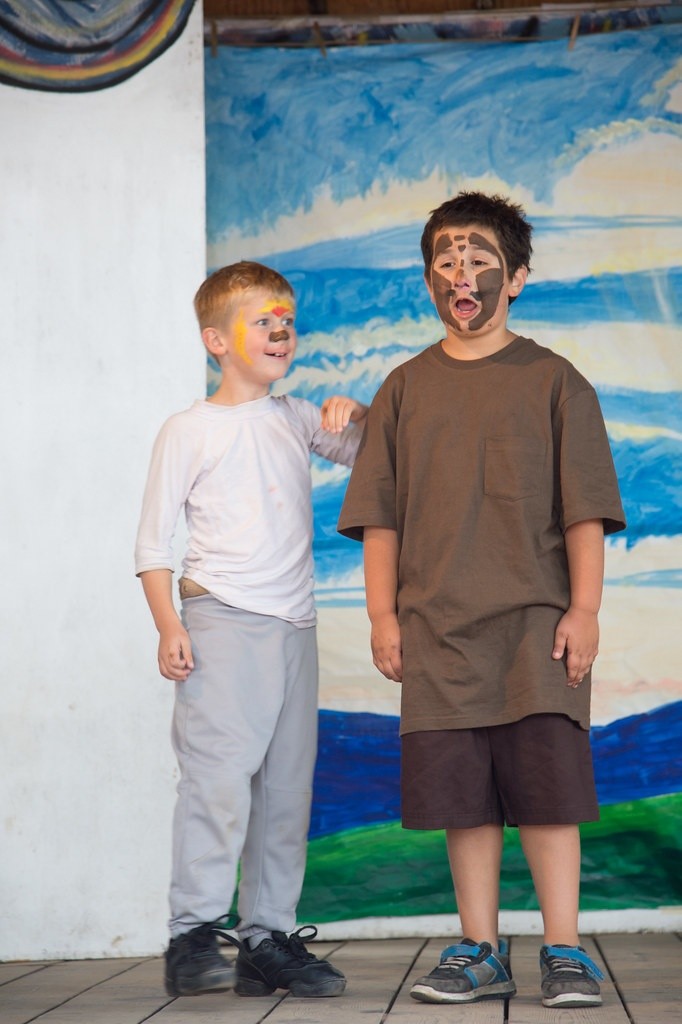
[539,943,605,1008]
[163,925,237,996]
[234,926,347,997]
[410,937,517,1004]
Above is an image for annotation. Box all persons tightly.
[136,263,368,998]
[336,193,627,1007]
